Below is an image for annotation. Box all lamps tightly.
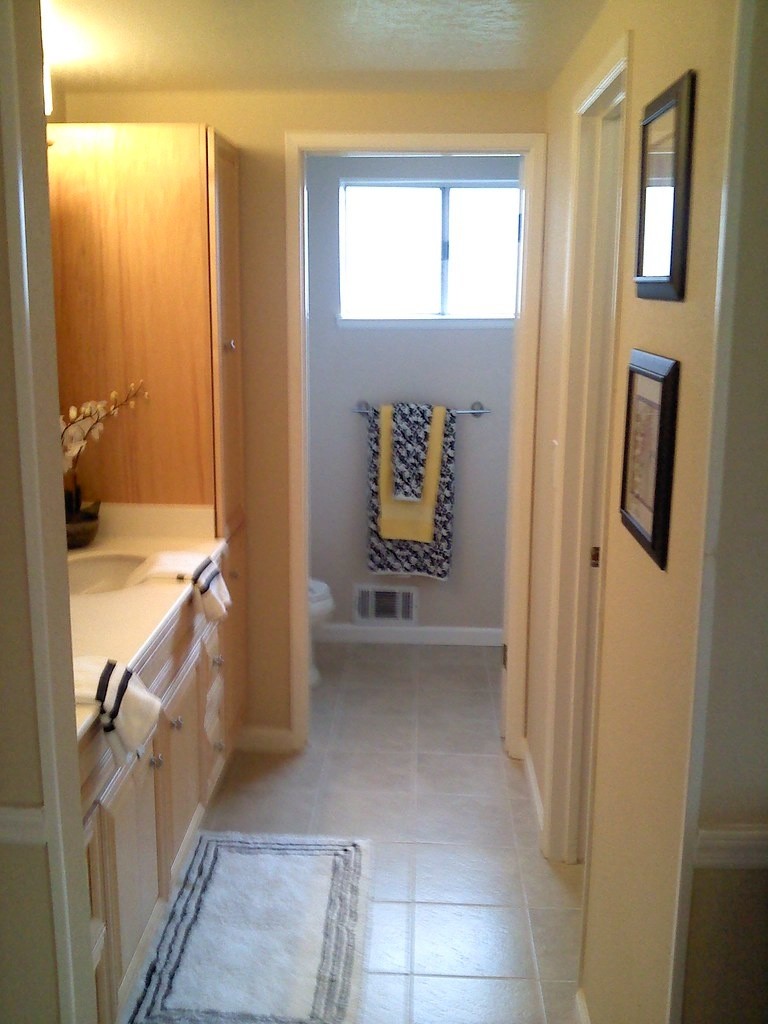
[39,15,60,114]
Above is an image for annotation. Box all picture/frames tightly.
[620,345,684,572]
[632,68,698,303]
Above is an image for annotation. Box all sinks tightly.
[65,547,157,604]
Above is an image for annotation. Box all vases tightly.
[65,500,103,548]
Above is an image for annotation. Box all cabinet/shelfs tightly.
[52,122,248,753]
[76,550,236,1024]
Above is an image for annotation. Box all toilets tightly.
[309,578,338,689]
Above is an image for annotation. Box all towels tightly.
[70,655,171,762]
[376,401,447,545]
[365,405,458,583]
[125,546,237,622]
[389,399,435,504]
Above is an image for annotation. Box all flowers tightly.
[57,376,151,509]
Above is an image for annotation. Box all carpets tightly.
[117,828,380,1022]
